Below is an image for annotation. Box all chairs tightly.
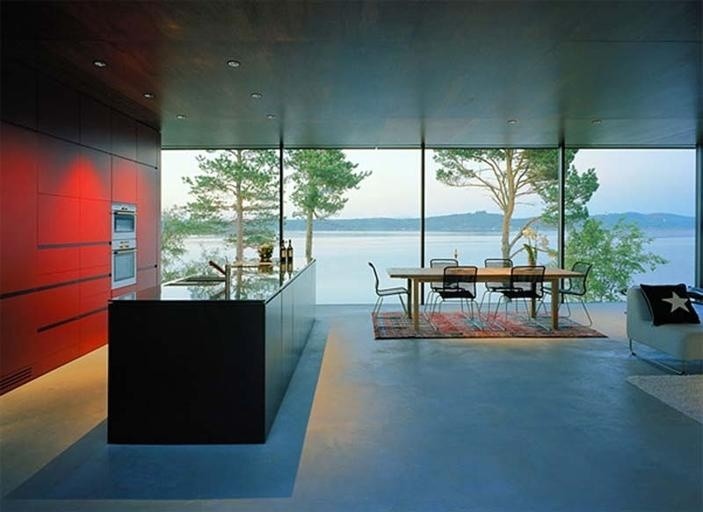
[368,258,594,332]
[626,287,703,375]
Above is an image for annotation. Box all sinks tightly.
[163,282,220,285]
[180,275,233,281]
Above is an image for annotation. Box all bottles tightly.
[279,239,293,263]
[280,263,293,280]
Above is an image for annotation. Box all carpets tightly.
[624,373,702,423]
[370,311,608,339]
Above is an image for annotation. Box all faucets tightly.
[208,255,231,295]
[209,281,231,300]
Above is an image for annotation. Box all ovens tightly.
[111,202,137,291]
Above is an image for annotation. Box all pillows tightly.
[639,284,701,326]
